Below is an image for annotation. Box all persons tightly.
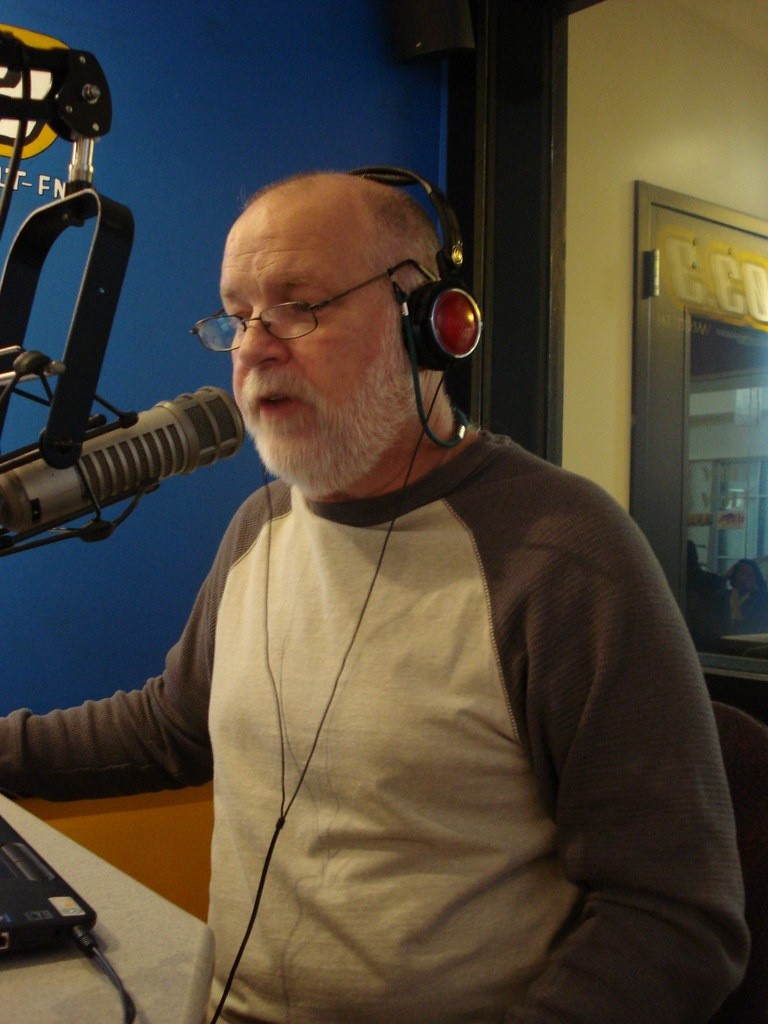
[0,174,751,1024]
[684,542,768,638]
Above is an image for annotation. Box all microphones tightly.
[0,386,241,544]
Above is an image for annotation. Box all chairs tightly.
[712,699,768,1024]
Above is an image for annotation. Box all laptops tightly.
[0,815,97,957]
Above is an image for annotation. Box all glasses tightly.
[189,257,436,352]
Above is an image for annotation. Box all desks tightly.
[0,790,216,1024]
[720,633,768,656]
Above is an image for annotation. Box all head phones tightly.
[351,163,484,370]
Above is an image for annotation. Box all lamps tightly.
[392,0,476,70]
